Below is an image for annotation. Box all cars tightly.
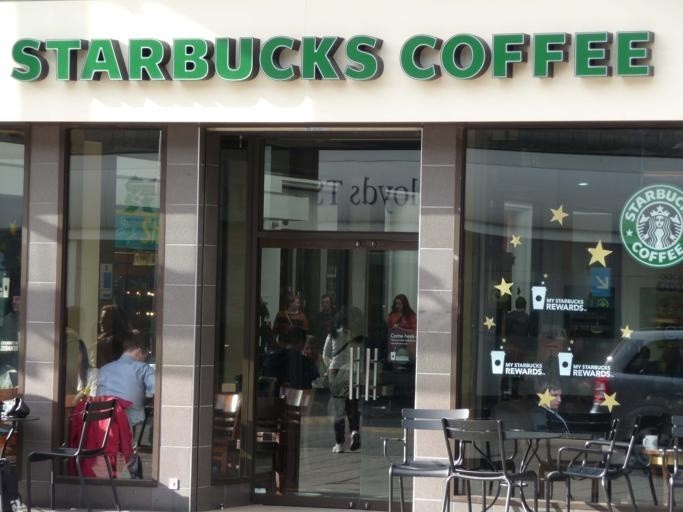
[549,328,683,476]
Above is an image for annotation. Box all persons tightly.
[257,291,416,493]
[500,296,538,404]
[0,288,20,386]
[77,338,99,397]
[95,335,156,453]
[531,378,574,433]
[638,207,681,249]
[89,304,124,369]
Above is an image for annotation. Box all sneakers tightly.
[349,430,361,451]
[331,441,344,453]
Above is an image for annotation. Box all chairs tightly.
[212,376,315,477]
[440,417,533,512]
[26,399,120,511]
[380,409,471,512]
[544,414,682,512]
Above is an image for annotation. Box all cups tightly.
[642,435,658,452]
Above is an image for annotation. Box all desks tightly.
[503,431,563,501]
[0,419,39,458]
